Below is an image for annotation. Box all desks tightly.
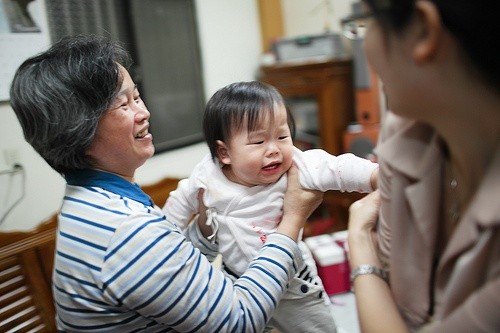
[261,54,356,158]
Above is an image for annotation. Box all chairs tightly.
[0,170,192,333]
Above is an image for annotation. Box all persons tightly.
[9,33,322,333]
[161,78,382,333]
[340,0,500,333]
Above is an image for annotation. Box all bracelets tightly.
[348,264,390,294]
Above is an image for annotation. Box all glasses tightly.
[339,1,414,40]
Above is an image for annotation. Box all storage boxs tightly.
[306,229,350,298]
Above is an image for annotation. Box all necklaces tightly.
[449,176,459,189]
